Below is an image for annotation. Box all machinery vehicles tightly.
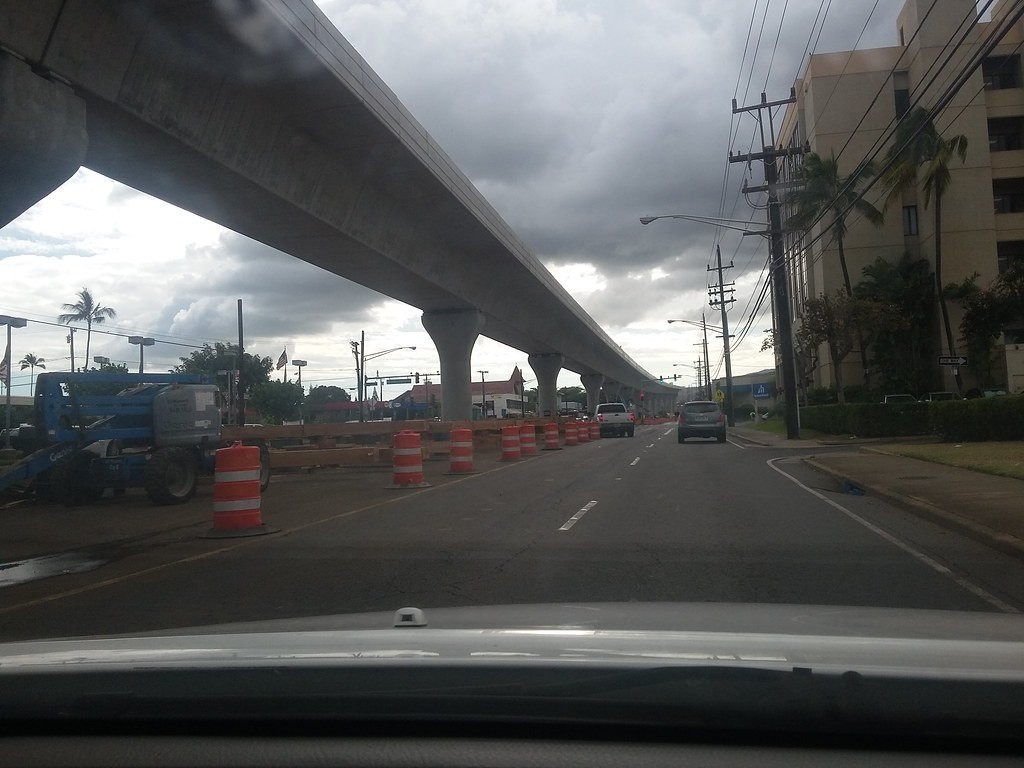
[28,366,269,507]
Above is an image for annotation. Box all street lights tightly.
[218,370,240,423]
[129,336,155,386]
[668,319,736,427]
[359,347,417,422]
[0,315,28,449]
[478,371,489,419]
[292,360,307,424]
[641,215,800,441]
[94,356,110,370]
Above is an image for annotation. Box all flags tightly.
[277,351,287,370]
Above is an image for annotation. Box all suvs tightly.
[677,400,727,444]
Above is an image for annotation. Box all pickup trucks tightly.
[593,403,634,438]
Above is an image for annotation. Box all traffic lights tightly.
[660,376,662,383]
